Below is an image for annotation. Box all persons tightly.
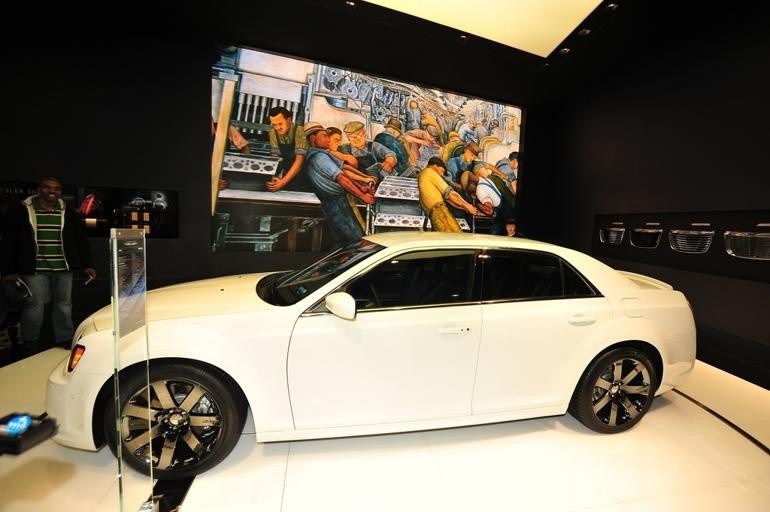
[4,177,97,354]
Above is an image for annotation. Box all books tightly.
[16,277,32,298]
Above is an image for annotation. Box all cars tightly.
[39,226,705,482]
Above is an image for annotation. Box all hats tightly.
[303,121,323,137]
[384,119,402,133]
[344,121,365,133]
[468,142,480,155]
